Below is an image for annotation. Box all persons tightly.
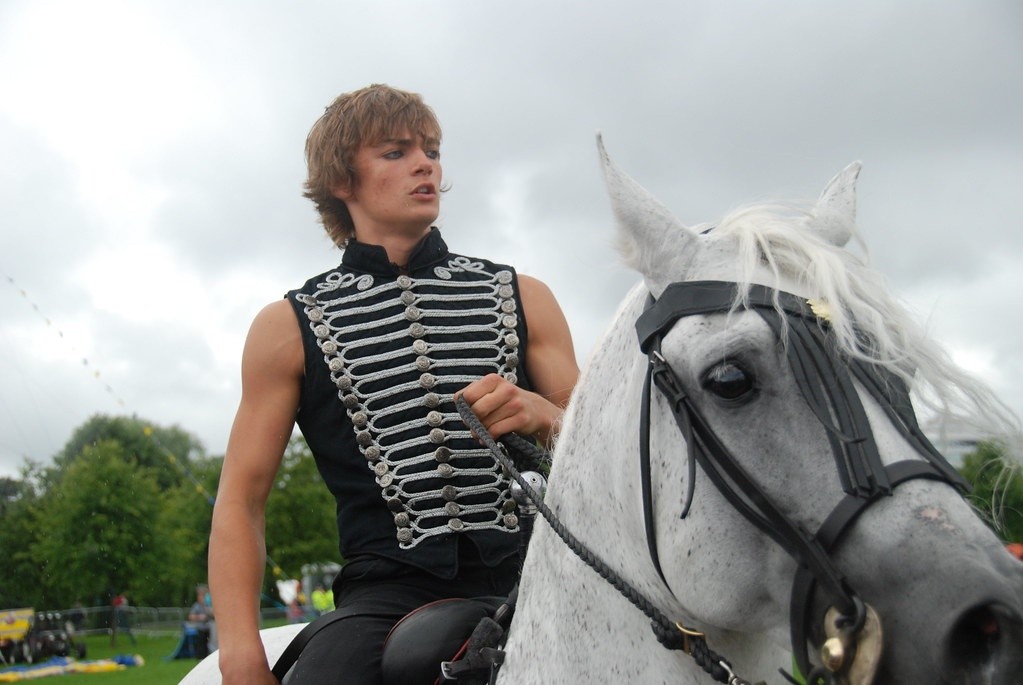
[287,600,309,624]
[207,84,580,685]
[187,584,216,659]
[311,580,335,617]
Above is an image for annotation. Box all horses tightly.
[178,124,1023,685]
